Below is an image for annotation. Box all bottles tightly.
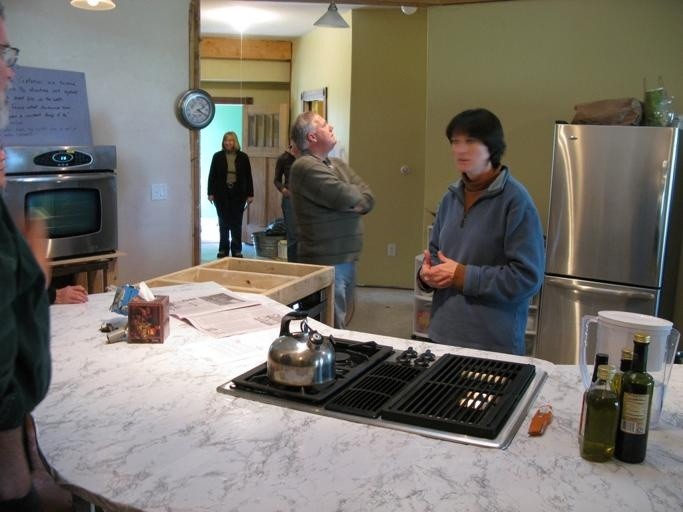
[576,333,656,464]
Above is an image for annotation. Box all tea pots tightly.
[576,309,682,430]
[266,309,333,390]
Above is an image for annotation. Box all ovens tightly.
[3,144,119,260]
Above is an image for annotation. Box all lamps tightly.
[69,0,119,13]
[311,0,355,32]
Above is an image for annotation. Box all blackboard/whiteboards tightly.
[0,65,93,148]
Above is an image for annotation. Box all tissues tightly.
[126,279,170,345]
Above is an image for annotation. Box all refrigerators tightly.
[529,122,683,365]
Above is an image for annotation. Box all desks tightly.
[43,250,129,295]
[31,281,683,511]
[129,254,338,326]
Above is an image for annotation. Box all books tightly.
[167,289,286,340]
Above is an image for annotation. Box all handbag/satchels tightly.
[643,75,671,127]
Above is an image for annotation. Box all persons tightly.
[274,137,301,222]
[0,5,51,512]
[208,132,254,258]
[416,108,546,356]
[1,145,88,305]
[289,112,375,329]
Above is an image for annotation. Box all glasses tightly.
[2,44,21,69]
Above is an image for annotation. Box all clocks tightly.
[174,87,216,130]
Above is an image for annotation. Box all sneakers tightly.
[217,249,228,258]
[232,250,243,257]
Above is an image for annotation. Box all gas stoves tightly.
[214,333,548,451]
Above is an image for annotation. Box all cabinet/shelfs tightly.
[408,250,545,349]
